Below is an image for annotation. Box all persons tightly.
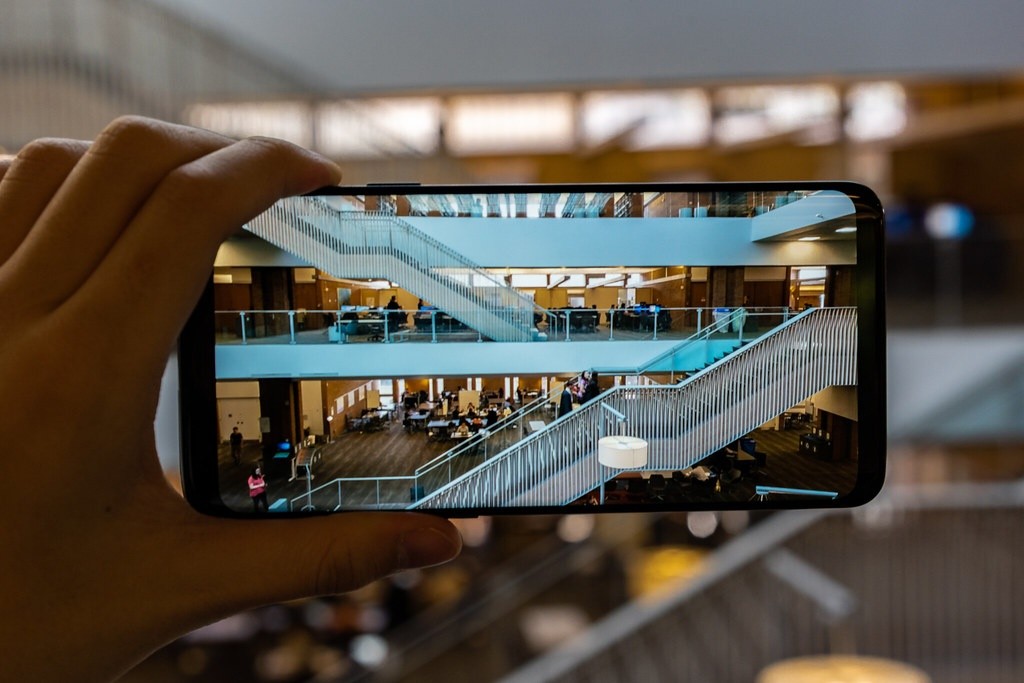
[0,114,460,683]
[230,287,672,512]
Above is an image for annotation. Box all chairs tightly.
[647,471,690,498]
[367,324,384,342]
[344,405,399,434]
[336,323,359,344]
[523,427,529,436]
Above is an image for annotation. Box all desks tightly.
[623,313,665,332]
[641,466,692,496]
[403,389,545,452]
[550,312,600,333]
[364,403,397,426]
[334,320,389,342]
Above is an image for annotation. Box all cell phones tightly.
[179,180,886,520]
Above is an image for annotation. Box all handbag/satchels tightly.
[578,397,581,403]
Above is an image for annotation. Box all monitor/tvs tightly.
[278,443,290,450]
[340,305,370,313]
[634,306,642,314]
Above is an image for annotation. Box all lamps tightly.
[598,419,647,469]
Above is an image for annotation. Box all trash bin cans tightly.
[711,309,731,334]
[743,310,760,333]
